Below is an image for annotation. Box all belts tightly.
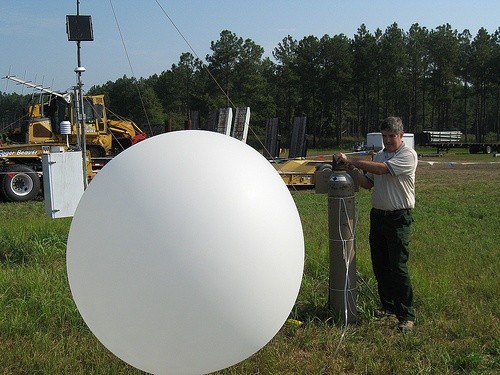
[371,208,411,216]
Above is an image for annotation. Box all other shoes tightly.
[397,318,415,331]
[374,308,392,318]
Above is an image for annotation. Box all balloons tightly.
[66,130,305,375]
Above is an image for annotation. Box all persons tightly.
[334,116,419,332]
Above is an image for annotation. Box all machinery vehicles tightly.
[0,95,135,203]
